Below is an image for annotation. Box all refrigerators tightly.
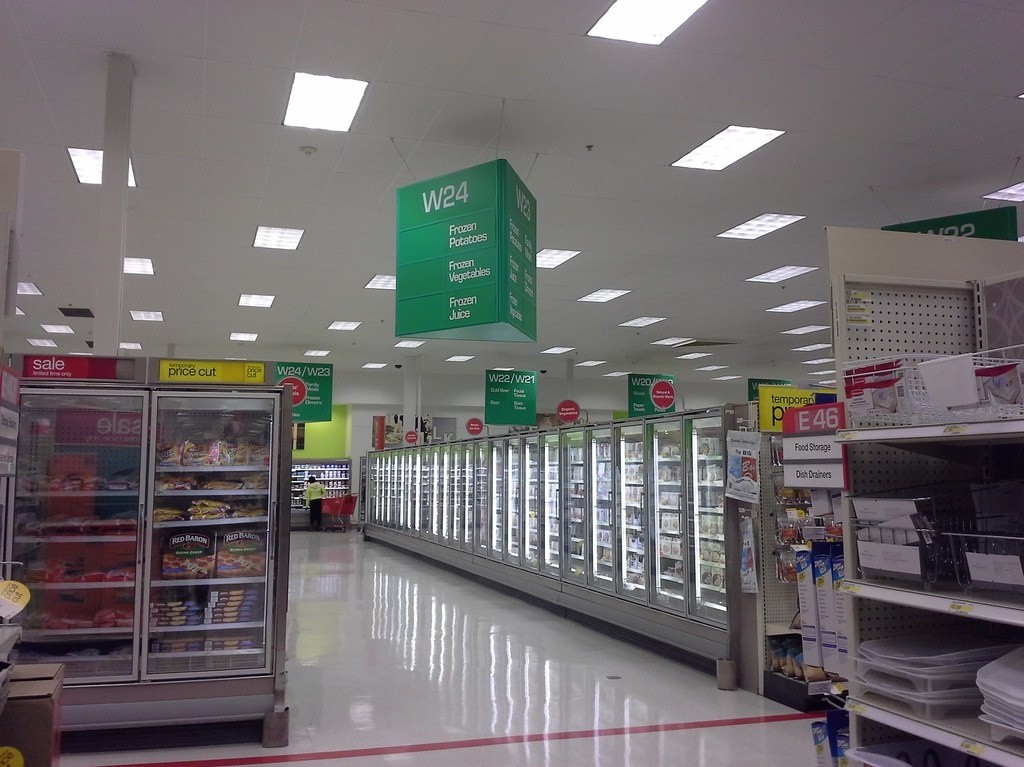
[360,404,746,660]
[12,382,292,730]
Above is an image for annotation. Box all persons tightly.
[306,476,327,532]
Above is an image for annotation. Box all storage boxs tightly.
[796,540,849,677]
[809,488,843,524]
[811,709,850,767]
[0,663,65,767]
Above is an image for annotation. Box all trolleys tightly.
[319,493,361,532]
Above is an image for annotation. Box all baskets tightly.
[842,481,1024,594]
[842,343,1024,431]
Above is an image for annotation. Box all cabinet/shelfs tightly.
[826,225,1024,767]
[6,380,293,749]
